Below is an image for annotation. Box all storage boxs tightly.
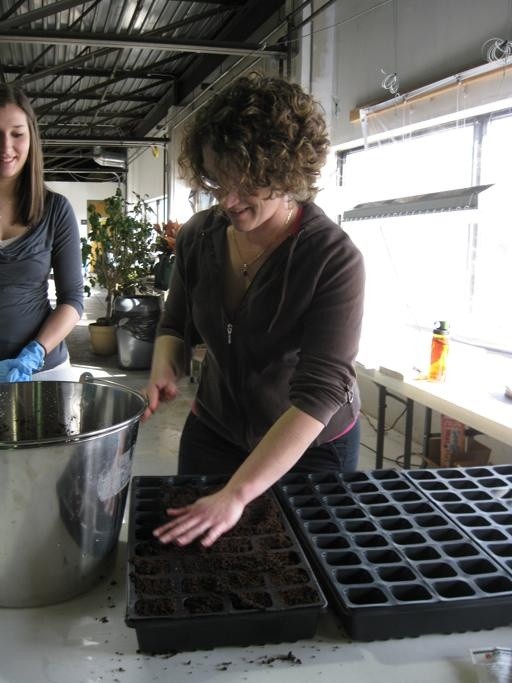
[419,430,491,467]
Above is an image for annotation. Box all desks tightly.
[357,345,511,468]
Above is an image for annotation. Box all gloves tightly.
[0,340,45,382]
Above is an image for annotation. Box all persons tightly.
[0,81,84,385]
[132,74,362,550]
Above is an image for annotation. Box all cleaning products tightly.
[428,319,451,380]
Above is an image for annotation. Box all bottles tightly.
[430,320,449,382]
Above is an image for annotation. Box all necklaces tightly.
[233,209,294,275]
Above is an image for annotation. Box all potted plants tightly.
[82,187,182,356]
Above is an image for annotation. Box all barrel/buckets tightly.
[116,294,162,370]
[0,373,150,609]
